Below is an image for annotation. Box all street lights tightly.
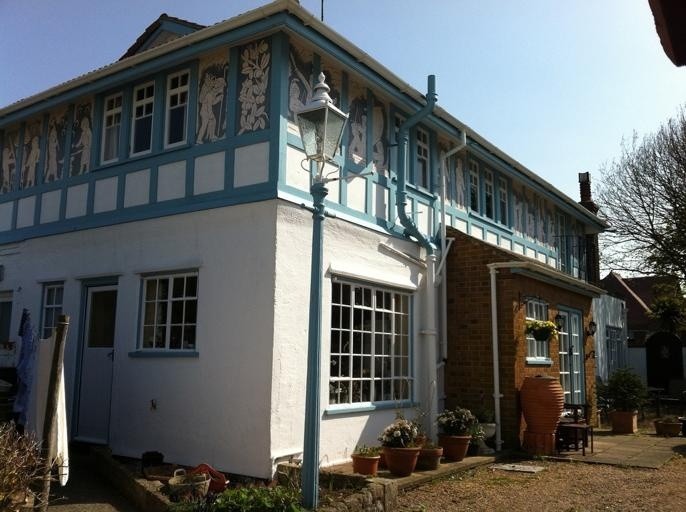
[295,70,350,512]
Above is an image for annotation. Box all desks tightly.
[559,425,595,456]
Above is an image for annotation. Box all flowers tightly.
[525,320,557,335]
[376,419,418,448]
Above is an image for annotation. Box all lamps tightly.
[555,314,565,332]
[587,321,596,337]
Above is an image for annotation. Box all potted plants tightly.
[654,416,683,437]
[606,364,643,434]
[434,406,475,461]
[350,443,382,478]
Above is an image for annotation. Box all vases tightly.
[377,451,387,469]
[528,328,551,341]
[417,446,443,470]
[382,445,422,477]
